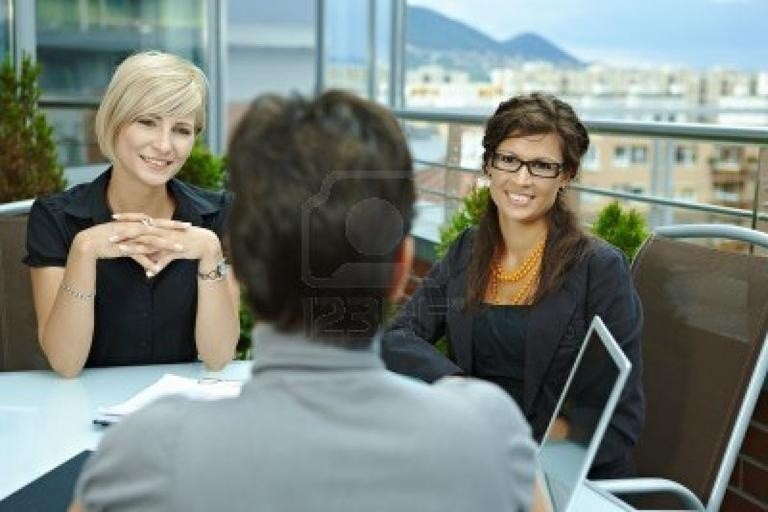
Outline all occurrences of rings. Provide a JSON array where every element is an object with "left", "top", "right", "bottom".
[{"left": 142, "top": 216, "right": 153, "bottom": 227}]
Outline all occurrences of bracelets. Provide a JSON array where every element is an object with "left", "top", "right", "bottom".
[
  {"left": 194, "top": 258, "right": 229, "bottom": 282},
  {"left": 55, "top": 282, "right": 95, "bottom": 303}
]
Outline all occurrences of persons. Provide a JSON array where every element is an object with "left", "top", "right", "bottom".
[
  {"left": 57, "top": 85, "right": 562, "bottom": 509},
  {"left": 17, "top": 49, "right": 242, "bottom": 384},
  {"left": 376, "top": 91, "right": 650, "bottom": 486}
]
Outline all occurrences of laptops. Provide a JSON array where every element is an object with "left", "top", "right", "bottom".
[{"left": 537, "top": 313, "right": 634, "bottom": 512}]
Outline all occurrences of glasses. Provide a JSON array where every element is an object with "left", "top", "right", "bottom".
[{"left": 487, "top": 151, "right": 569, "bottom": 179}]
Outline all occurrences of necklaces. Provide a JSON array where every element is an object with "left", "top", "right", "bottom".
[{"left": 488, "top": 228, "right": 549, "bottom": 308}]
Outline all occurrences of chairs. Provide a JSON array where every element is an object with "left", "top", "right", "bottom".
[{"left": 582, "top": 218, "right": 767, "bottom": 510}]
